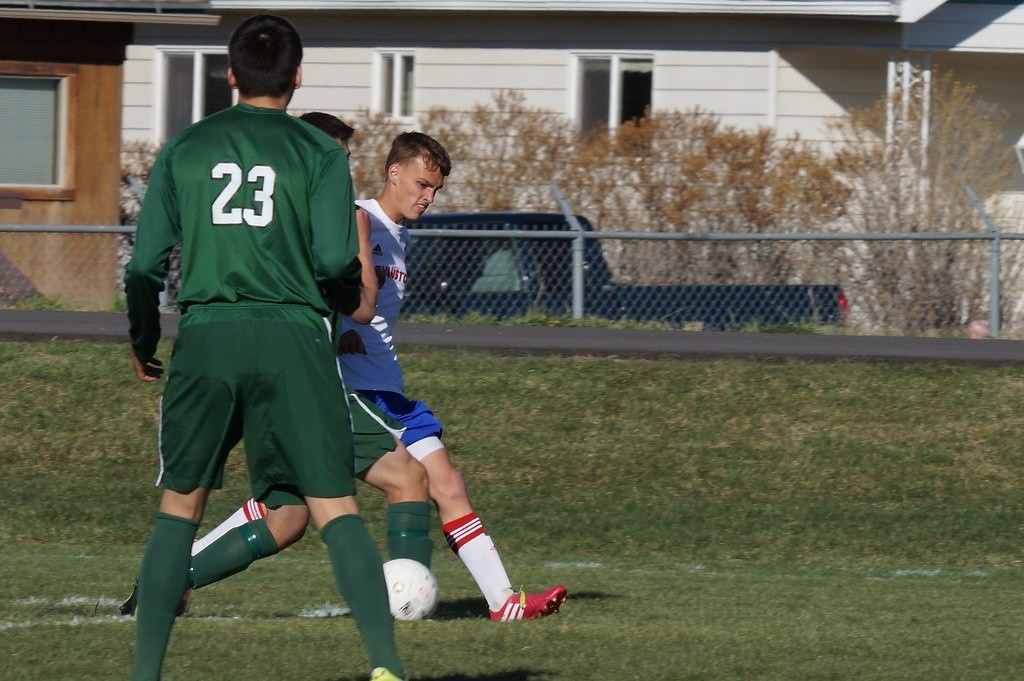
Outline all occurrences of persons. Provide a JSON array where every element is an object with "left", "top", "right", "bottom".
[
  {"left": 120, "top": 13, "right": 405, "bottom": 681},
  {"left": 121, "top": 112, "right": 436, "bottom": 622},
  {"left": 178, "top": 133, "right": 569, "bottom": 622}
]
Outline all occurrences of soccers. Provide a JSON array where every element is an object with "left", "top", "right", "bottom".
[{"left": 383, "top": 558, "right": 438, "bottom": 621}]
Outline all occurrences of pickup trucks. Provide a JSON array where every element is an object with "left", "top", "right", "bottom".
[{"left": 402, "top": 211, "right": 849, "bottom": 328}]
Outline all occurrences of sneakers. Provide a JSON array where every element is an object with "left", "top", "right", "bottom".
[
  {"left": 488, "top": 584, "right": 568, "bottom": 622},
  {"left": 119, "top": 579, "right": 189, "bottom": 616},
  {"left": 371, "top": 667, "right": 400, "bottom": 680}
]
